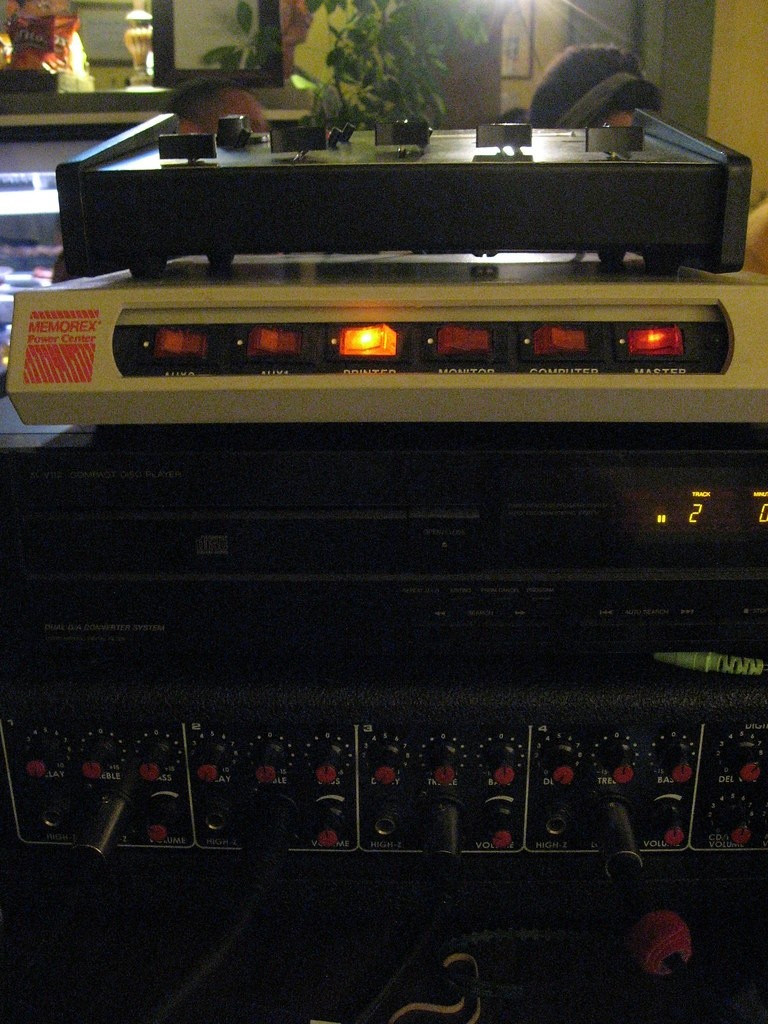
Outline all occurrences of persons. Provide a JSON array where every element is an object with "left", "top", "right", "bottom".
[
  {"left": 0, "top": 0, "right": 97, "bottom": 93},
  {"left": 531, "top": 44, "right": 662, "bottom": 129},
  {"left": 165, "top": 74, "right": 273, "bottom": 133}
]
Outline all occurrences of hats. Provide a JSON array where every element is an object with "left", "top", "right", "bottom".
[{"left": 530, "top": 44, "right": 661, "bottom": 131}]
